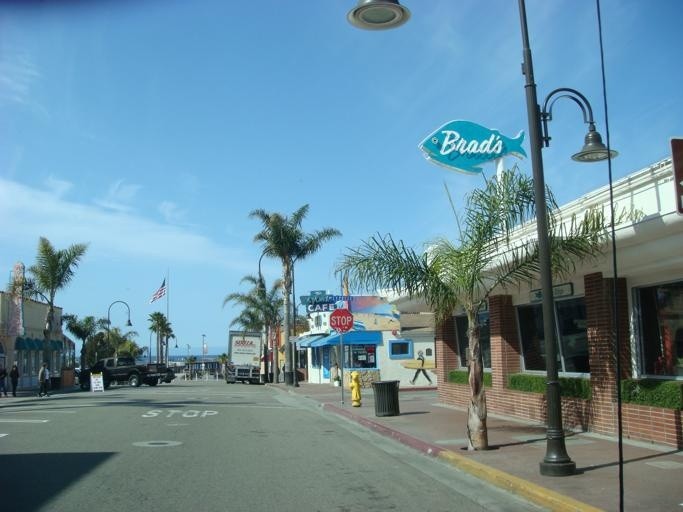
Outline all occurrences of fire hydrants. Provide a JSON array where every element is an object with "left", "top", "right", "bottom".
[{"left": 349, "top": 371, "right": 360, "bottom": 407}]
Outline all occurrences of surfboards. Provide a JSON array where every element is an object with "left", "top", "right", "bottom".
[{"left": 400, "top": 360, "right": 436, "bottom": 369}]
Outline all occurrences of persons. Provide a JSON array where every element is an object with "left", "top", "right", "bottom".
[
  {"left": 408, "top": 351, "right": 432, "bottom": 387},
  {"left": 8, "top": 365, "right": 19, "bottom": 397},
  {"left": 0, "top": 363, "right": 9, "bottom": 399},
  {"left": 37, "top": 362, "right": 51, "bottom": 397}
]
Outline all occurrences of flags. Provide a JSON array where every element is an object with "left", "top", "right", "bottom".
[{"left": 148, "top": 277, "right": 166, "bottom": 305}]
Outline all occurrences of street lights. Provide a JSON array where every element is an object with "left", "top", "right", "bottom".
[
  {"left": 345, "top": 0, "right": 617, "bottom": 475},
  {"left": 106, "top": 301, "right": 132, "bottom": 357},
  {"left": 165, "top": 334, "right": 177, "bottom": 364}
]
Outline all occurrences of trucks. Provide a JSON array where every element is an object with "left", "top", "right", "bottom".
[{"left": 222, "top": 331, "right": 261, "bottom": 384}]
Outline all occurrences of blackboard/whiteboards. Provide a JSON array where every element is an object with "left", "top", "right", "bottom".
[{"left": 91, "top": 373, "right": 104, "bottom": 390}]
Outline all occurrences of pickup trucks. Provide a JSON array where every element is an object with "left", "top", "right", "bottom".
[{"left": 78, "top": 357, "right": 160, "bottom": 391}]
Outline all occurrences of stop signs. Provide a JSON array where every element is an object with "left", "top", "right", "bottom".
[{"left": 329, "top": 309, "right": 352, "bottom": 333}]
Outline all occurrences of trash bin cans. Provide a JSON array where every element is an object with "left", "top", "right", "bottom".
[
  {"left": 371, "top": 380, "right": 400, "bottom": 416},
  {"left": 286, "top": 371, "right": 298, "bottom": 386},
  {"left": 269, "top": 373, "right": 277, "bottom": 382},
  {"left": 51, "top": 376, "right": 61, "bottom": 391},
  {"left": 261, "top": 374, "right": 268, "bottom": 383}
]
